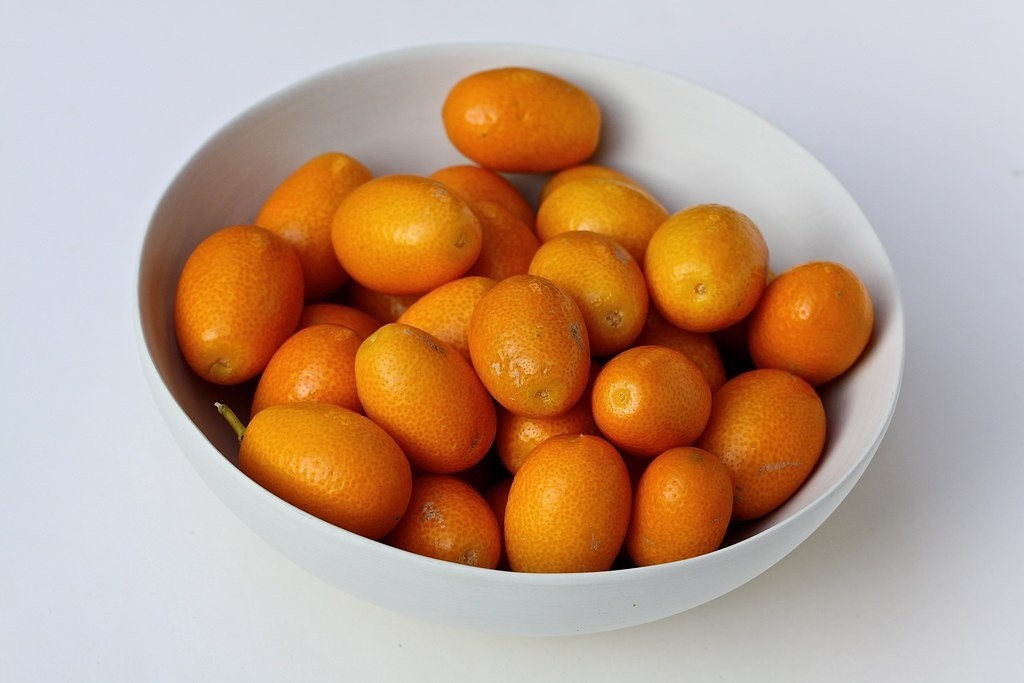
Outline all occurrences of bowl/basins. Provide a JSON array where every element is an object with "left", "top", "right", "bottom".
[{"left": 132, "top": 40, "right": 905, "bottom": 637}]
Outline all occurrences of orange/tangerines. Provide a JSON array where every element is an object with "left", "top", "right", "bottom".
[{"left": 177, "top": 67, "right": 872, "bottom": 573}]
